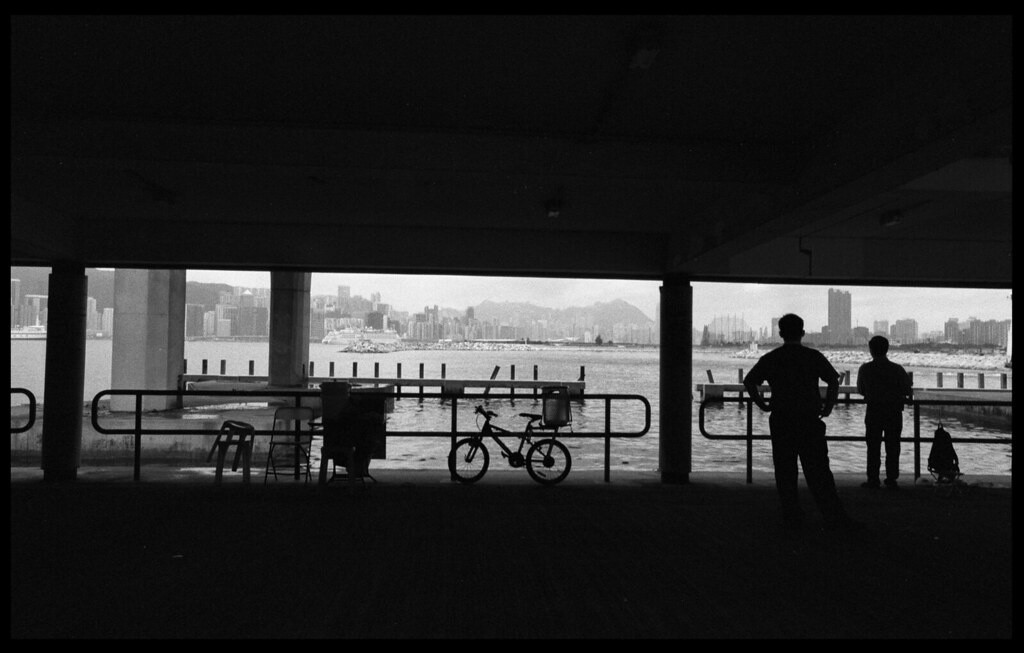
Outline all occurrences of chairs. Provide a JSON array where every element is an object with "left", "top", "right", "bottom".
[{"left": 264, "top": 407, "right": 315, "bottom": 486}]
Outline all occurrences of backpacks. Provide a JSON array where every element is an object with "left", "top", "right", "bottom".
[{"left": 927, "top": 422, "right": 960, "bottom": 483}]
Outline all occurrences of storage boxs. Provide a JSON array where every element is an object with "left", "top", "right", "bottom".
[{"left": 542, "top": 386, "right": 571, "bottom": 427}]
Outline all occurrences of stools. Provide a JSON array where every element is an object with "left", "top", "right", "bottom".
[{"left": 206, "top": 421, "right": 254, "bottom": 487}]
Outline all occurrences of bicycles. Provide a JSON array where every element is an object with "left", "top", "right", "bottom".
[{"left": 447, "top": 404, "right": 572, "bottom": 485}]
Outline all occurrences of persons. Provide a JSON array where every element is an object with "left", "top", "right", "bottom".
[
  {"left": 856, "top": 336, "right": 912, "bottom": 487},
  {"left": 744, "top": 313, "right": 840, "bottom": 500}
]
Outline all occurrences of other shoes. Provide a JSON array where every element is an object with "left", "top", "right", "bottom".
[
  {"left": 827, "top": 516, "right": 862, "bottom": 530},
  {"left": 861, "top": 481, "right": 880, "bottom": 489},
  {"left": 785, "top": 506, "right": 805, "bottom": 532},
  {"left": 884, "top": 478, "right": 898, "bottom": 487}
]
